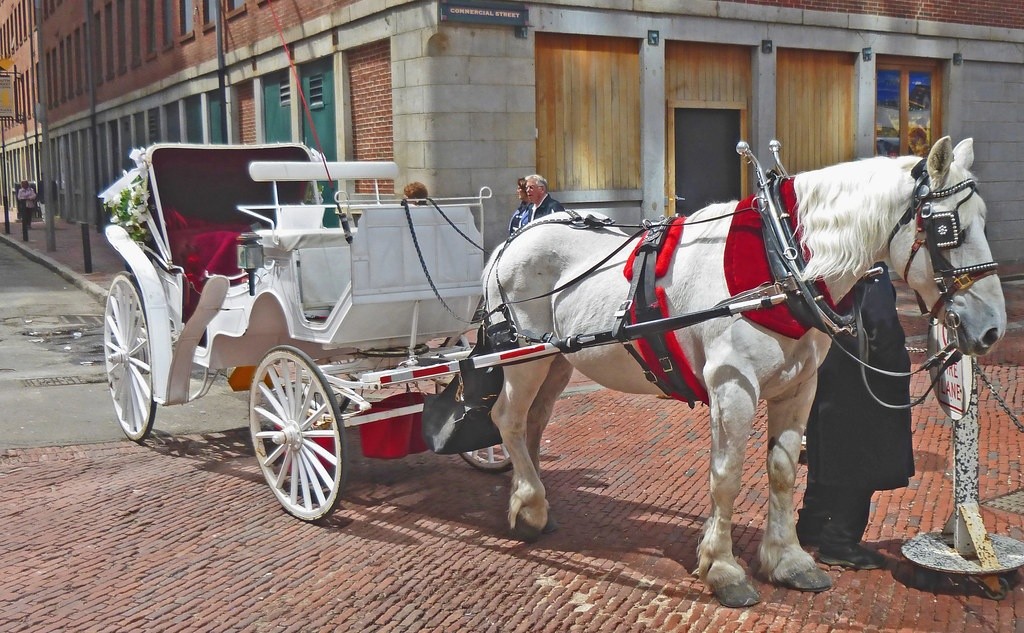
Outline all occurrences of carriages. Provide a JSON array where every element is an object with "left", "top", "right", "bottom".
[{"left": 103, "top": 134, "right": 1008, "bottom": 610}]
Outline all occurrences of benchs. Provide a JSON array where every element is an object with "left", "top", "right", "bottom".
[
  {"left": 147, "top": 140, "right": 318, "bottom": 325},
  {"left": 236, "top": 160, "right": 404, "bottom": 311}
]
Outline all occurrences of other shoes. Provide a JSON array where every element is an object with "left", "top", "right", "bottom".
[{"left": 816, "top": 539, "right": 887, "bottom": 570}]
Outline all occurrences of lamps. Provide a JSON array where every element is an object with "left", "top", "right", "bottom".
[{"left": 424, "top": 30, "right": 451, "bottom": 58}]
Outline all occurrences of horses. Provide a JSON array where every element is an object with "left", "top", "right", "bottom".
[{"left": 482, "top": 136, "right": 1007, "bottom": 608}]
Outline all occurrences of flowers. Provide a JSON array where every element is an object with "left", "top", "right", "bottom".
[{"left": 104, "top": 148, "right": 154, "bottom": 243}]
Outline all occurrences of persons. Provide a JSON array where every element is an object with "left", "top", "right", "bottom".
[
  {"left": 508, "top": 174, "right": 565, "bottom": 237},
  {"left": 794, "top": 260, "right": 914, "bottom": 565},
  {"left": 18, "top": 180, "right": 37, "bottom": 241}
]
